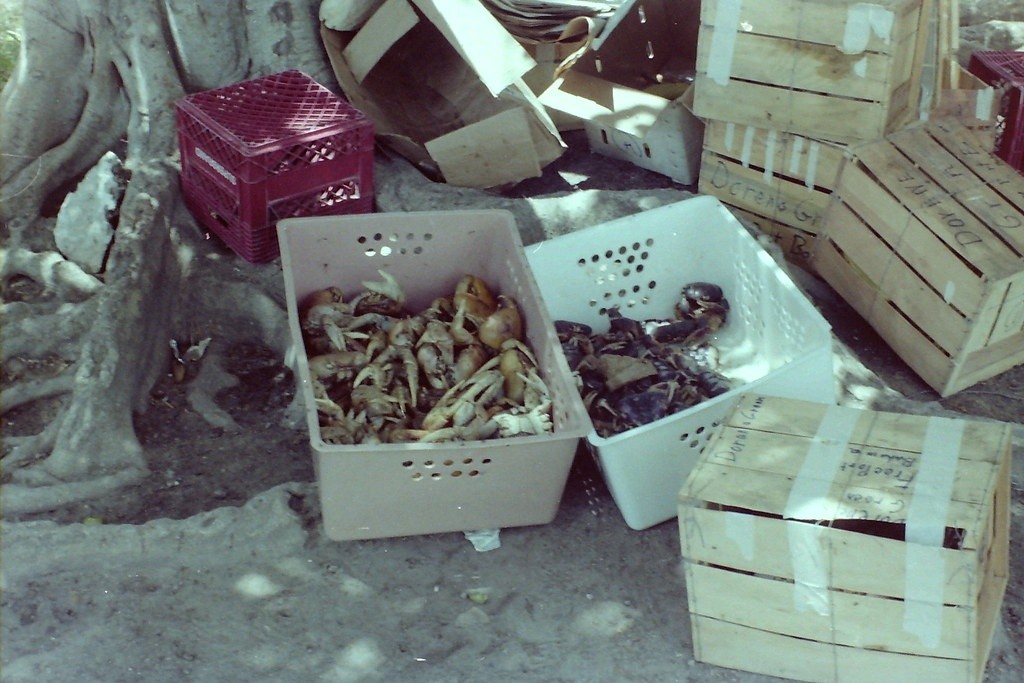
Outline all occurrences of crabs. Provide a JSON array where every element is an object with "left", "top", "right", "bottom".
[
  {"left": 298, "top": 266, "right": 551, "bottom": 441},
  {"left": 553, "top": 282, "right": 747, "bottom": 437}
]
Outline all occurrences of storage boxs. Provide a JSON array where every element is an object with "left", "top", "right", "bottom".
[
  {"left": 274, "top": 208, "right": 592, "bottom": 543},
  {"left": 172, "top": 67, "right": 375, "bottom": 266},
  {"left": 320, "top": 1, "right": 570, "bottom": 194},
  {"left": 692, "top": 2, "right": 929, "bottom": 141},
  {"left": 696, "top": 117, "right": 849, "bottom": 279},
  {"left": 523, "top": 193, "right": 839, "bottom": 531},
  {"left": 812, "top": 115, "right": 1024, "bottom": 398},
  {"left": 536, "top": 0, "right": 702, "bottom": 185},
  {"left": 675, "top": 393, "right": 1013, "bottom": 682},
  {"left": 968, "top": 50, "right": 1024, "bottom": 178}
]
